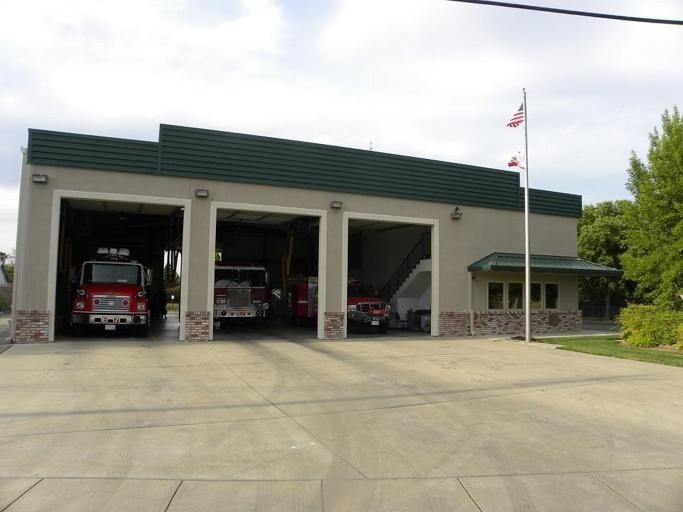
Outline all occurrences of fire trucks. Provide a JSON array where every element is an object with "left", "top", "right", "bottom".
[
  {"left": 67, "top": 247, "right": 166, "bottom": 336},
  {"left": 288, "top": 274, "right": 391, "bottom": 333},
  {"left": 214, "top": 260, "right": 270, "bottom": 319}
]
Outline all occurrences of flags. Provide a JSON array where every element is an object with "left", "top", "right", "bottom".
[{"left": 506, "top": 103, "right": 524, "bottom": 126}]
